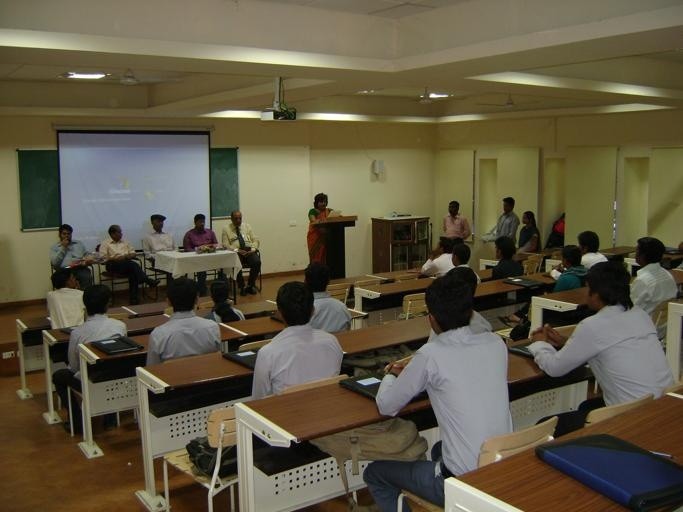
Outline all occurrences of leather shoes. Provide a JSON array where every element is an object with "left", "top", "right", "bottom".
[
  {"left": 248, "top": 285, "right": 256, "bottom": 294},
  {"left": 241, "top": 287, "right": 247, "bottom": 296},
  {"left": 148, "top": 279, "right": 160, "bottom": 287}
]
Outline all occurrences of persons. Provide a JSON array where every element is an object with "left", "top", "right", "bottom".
[
  {"left": 442, "top": 200, "right": 470, "bottom": 243},
  {"left": 221, "top": 210, "right": 261, "bottom": 296},
  {"left": 98, "top": 224, "right": 160, "bottom": 304},
  {"left": 204, "top": 281, "right": 245, "bottom": 322},
  {"left": 497, "top": 244, "right": 590, "bottom": 325},
  {"left": 575, "top": 230, "right": 609, "bottom": 271},
  {"left": 301, "top": 261, "right": 351, "bottom": 337},
  {"left": 49, "top": 223, "right": 91, "bottom": 291},
  {"left": 145, "top": 276, "right": 220, "bottom": 367},
  {"left": 491, "top": 236, "right": 524, "bottom": 280},
  {"left": 544, "top": 213, "right": 565, "bottom": 249},
  {"left": 444, "top": 244, "right": 481, "bottom": 287},
  {"left": 45, "top": 268, "right": 86, "bottom": 330},
  {"left": 141, "top": 215, "right": 177, "bottom": 263},
  {"left": 627, "top": 236, "right": 678, "bottom": 316},
  {"left": 362, "top": 274, "right": 514, "bottom": 511},
  {"left": 305, "top": 192, "right": 333, "bottom": 272},
  {"left": 422, "top": 235, "right": 456, "bottom": 279},
  {"left": 249, "top": 280, "right": 344, "bottom": 398},
  {"left": 50, "top": 285, "right": 128, "bottom": 434},
  {"left": 490, "top": 197, "right": 520, "bottom": 248},
  {"left": 182, "top": 214, "right": 218, "bottom": 283},
  {"left": 518, "top": 210, "right": 541, "bottom": 252},
  {"left": 526, "top": 260, "right": 678, "bottom": 441}
]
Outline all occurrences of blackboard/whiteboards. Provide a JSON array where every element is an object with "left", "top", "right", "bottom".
[{"left": 16, "top": 146, "right": 240, "bottom": 233}]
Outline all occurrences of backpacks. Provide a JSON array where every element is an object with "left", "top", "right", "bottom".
[
  {"left": 312, "top": 418, "right": 428, "bottom": 512},
  {"left": 188, "top": 436, "right": 239, "bottom": 476}
]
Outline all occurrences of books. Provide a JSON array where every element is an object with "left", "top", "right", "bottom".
[
  {"left": 508, "top": 341, "right": 534, "bottom": 357},
  {"left": 90, "top": 338, "right": 136, "bottom": 354},
  {"left": 338, "top": 372, "right": 387, "bottom": 399},
  {"left": 59, "top": 325, "right": 78, "bottom": 335},
  {"left": 221, "top": 347, "right": 260, "bottom": 369},
  {"left": 662, "top": 246, "right": 679, "bottom": 255},
  {"left": 500, "top": 275, "right": 542, "bottom": 289}
]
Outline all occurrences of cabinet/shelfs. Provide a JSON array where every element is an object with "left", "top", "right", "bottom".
[{"left": 371, "top": 217, "right": 433, "bottom": 273}]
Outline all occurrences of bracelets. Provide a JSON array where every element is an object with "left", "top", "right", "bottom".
[{"left": 388, "top": 372, "right": 397, "bottom": 377}]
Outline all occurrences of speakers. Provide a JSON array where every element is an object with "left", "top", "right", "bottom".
[{"left": 373, "top": 159, "right": 384, "bottom": 174}]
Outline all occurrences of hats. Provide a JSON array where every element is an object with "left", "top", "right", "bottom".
[{"left": 151, "top": 214, "right": 165, "bottom": 220}]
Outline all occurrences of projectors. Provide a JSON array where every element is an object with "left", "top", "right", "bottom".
[{"left": 260, "top": 110, "right": 297, "bottom": 122}]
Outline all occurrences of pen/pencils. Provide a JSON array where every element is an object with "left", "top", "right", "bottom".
[{"left": 650, "top": 450, "right": 673, "bottom": 458}]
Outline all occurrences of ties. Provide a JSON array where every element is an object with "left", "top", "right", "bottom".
[{"left": 236, "top": 227, "right": 246, "bottom": 250}]
